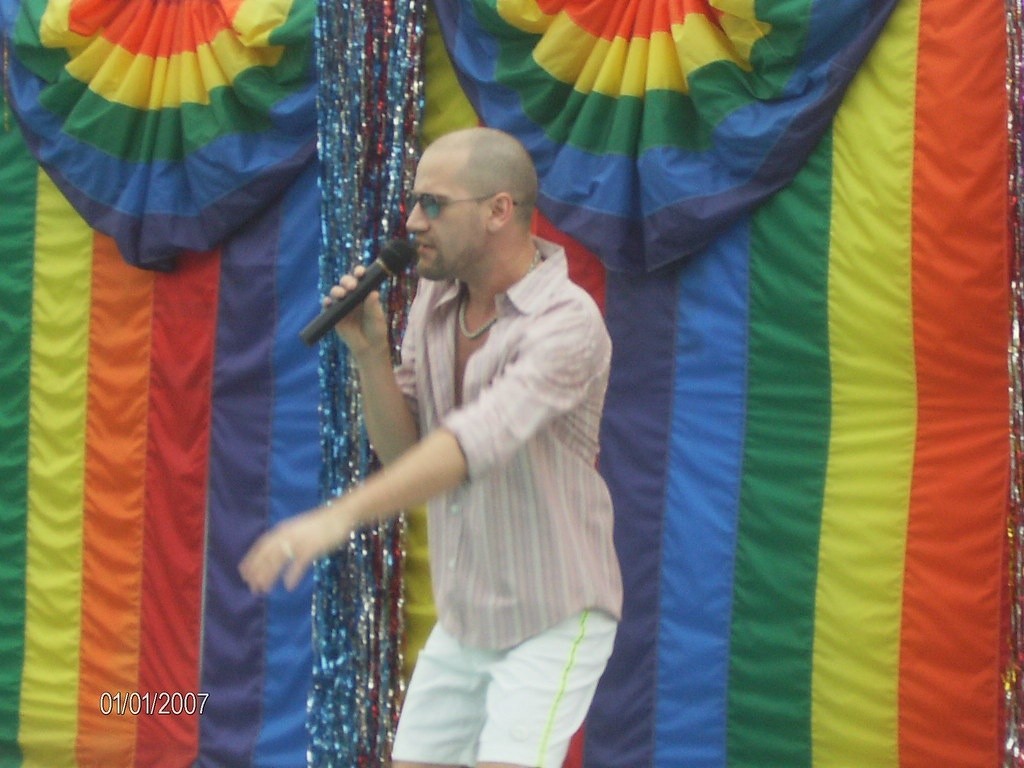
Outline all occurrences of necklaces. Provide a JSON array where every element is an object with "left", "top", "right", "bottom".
[{"left": 459, "top": 249, "right": 541, "bottom": 339}]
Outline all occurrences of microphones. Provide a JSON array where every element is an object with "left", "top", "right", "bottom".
[{"left": 300, "top": 241, "right": 412, "bottom": 346}]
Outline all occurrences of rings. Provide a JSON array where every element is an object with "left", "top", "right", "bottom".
[{"left": 281, "top": 545, "right": 292, "bottom": 560}]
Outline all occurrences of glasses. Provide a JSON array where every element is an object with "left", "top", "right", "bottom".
[{"left": 401, "top": 189, "right": 518, "bottom": 218}]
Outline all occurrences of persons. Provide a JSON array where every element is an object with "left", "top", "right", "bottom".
[{"left": 238, "top": 127, "right": 622, "bottom": 768}]
